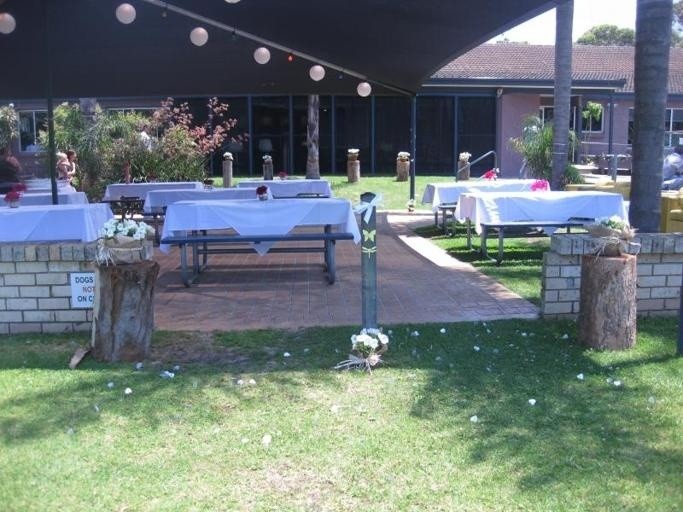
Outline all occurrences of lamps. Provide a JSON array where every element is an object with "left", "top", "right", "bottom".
[
  {"left": 0, "top": 10, "right": 16, "bottom": 39},
  {"left": 114, "top": 1, "right": 373, "bottom": 101}
]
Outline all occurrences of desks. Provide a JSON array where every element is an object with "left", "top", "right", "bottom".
[
  {"left": 423, "top": 179, "right": 629, "bottom": 264},
  {"left": 0, "top": 179, "right": 112, "bottom": 241},
  {"left": 104, "top": 180, "right": 361, "bottom": 282}
]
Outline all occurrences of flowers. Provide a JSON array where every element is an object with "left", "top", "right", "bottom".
[
  {"left": 333, "top": 327, "right": 392, "bottom": 377},
  {"left": 222, "top": 146, "right": 471, "bottom": 163}
]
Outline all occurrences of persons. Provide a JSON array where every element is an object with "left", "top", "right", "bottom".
[
  {"left": 67, "top": 149, "right": 77, "bottom": 185},
  {"left": 57, "top": 149, "right": 70, "bottom": 178},
  {"left": 663, "top": 146, "right": 683, "bottom": 190}
]
